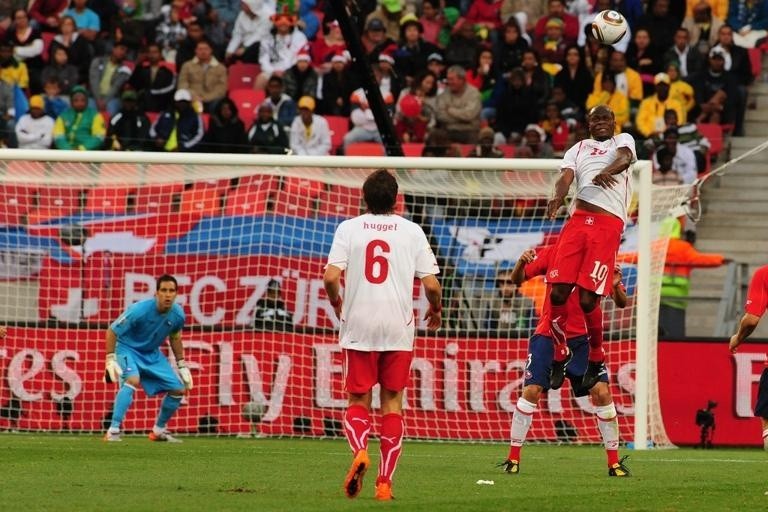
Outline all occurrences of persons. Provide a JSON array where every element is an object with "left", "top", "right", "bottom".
[
  {"left": 630, "top": 220, "right": 730, "bottom": 341},
  {"left": 486, "top": 270, "right": 537, "bottom": 340},
  {"left": 504, "top": 240, "right": 631, "bottom": 479},
  {"left": 0, "top": 2, "right": 767, "bottom": 232},
  {"left": 324, "top": 170, "right": 443, "bottom": 505},
  {"left": 104, "top": 271, "right": 194, "bottom": 443},
  {"left": 730, "top": 261, "right": 767, "bottom": 466},
  {"left": 255, "top": 279, "right": 292, "bottom": 327},
  {"left": 544, "top": 106, "right": 638, "bottom": 391}
]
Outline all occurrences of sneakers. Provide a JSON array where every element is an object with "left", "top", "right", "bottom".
[
  {"left": 505, "top": 459, "right": 520, "bottom": 473},
  {"left": 608, "top": 462, "right": 632, "bottom": 478},
  {"left": 148, "top": 429, "right": 183, "bottom": 443},
  {"left": 103, "top": 431, "right": 121, "bottom": 442},
  {"left": 581, "top": 359, "right": 605, "bottom": 390},
  {"left": 549, "top": 348, "right": 574, "bottom": 390},
  {"left": 374, "top": 483, "right": 394, "bottom": 501},
  {"left": 343, "top": 449, "right": 370, "bottom": 499}
]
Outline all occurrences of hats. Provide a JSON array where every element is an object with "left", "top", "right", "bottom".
[
  {"left": 295, "top": 46, "right": 313, "bottom": 63},
  {"left": 383, "top": 0, "right": 404, "bottom": 15},
  {"left": 367, "top": 18, "right": 386, "bottom": 32},
  {"left": 426, "top": 53, "right": 443, "bottom": 65},
  {"left": 298, "top": 96, "right": 317, "bottom": 112},
  {"left": 29, "top": 94, "right": 44, "bottom": 110},
  {"left": 378, "top": 45, "right": 398, "bottom": 66},
  {"left": 653, "top": 71, "right": 671, "bottom": 86},
  {"left": 174, "top": 88, "right": 191, "bottom": 102},
  {"left": 121, "top": 90, "right": 137, "bottom": 102},
  {"left": 331, "top": 49, "right": 347, "bottom": 65},
  {"left": 399, "top": 13, "right": 424, "bottom": 33}
]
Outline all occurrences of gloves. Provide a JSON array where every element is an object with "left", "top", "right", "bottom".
[
  {"left": 177, "top": 358, "right": 194, "bottom": 390},
  {"left": 102, "top": 352, "right": 123, "bottom": 384}
]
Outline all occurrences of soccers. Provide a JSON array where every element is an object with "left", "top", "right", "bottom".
[{"left": 590, "top": 9, "right": 627, "bottom": 45}]
